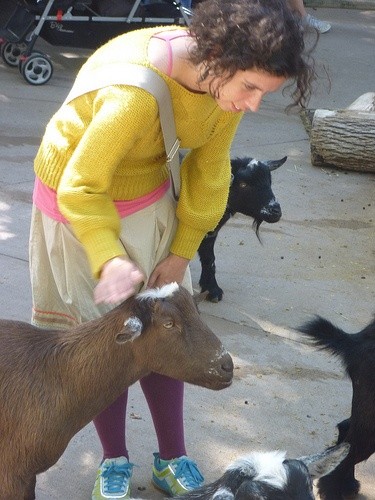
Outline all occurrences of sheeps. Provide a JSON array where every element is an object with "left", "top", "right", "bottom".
[
  {"left": 180, "top": 155, "right": 288, "bottom": 302},
  {"left": 163, "top": 441, "right": 351, "bottom": 500},
  {"left": 296, "top": 314, "right": 375, "bottom": 500},
  {"left": 0, "top": 282, "right": 234, "bottom": 500}
]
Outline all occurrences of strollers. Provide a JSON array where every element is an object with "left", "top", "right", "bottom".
[{"left": 1, "top": 0, "right": 202, "bottom": 86}]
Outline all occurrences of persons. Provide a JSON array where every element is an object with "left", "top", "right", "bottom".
[
  {"left": 284, "top": 0, "right": 330, "bottom": 33},
  {"left": 28, "top": 1, "right": 322, "bottom": 500}
]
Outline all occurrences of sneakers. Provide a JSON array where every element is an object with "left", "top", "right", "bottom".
[
  {"left": 92, "top": 456, "right": 135, "bottom": 500},
  {"left": 292, "top": 14, "right": 331, "bottom": 34},
  {"left": 151, "top": 448, "right": 205, "bottom": 498}
]
